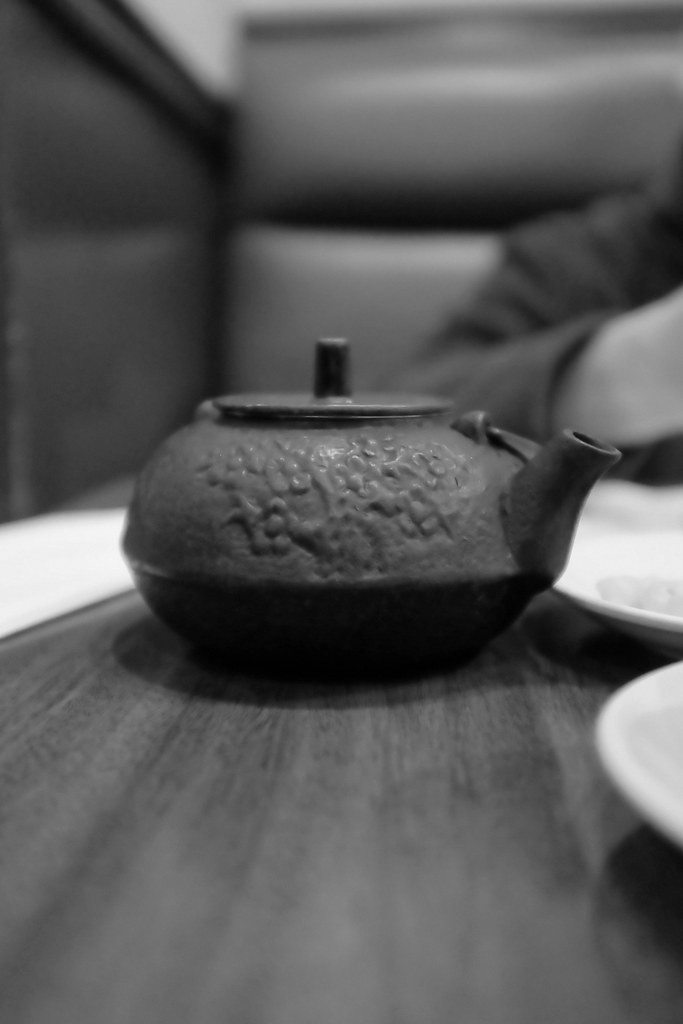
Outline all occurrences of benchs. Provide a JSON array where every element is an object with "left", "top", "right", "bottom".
[{"left": 227, "top": 16, "right": 682, "bottom": 405}]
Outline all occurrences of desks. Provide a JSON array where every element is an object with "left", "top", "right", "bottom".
[{"left": 0, "top": 471, "right": 683, "bottom": 1024}]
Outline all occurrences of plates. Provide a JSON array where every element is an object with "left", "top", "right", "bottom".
[
  {"left": 599, "top": 658, "right": 683, "bottom": 854},
  {"left": 547, "top": 477, "right": 683, "bottom": 656}
]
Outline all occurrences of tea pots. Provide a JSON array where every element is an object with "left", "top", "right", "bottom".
[{"left": 121, "top": 335, "right": 620, "bottom": 677}]
